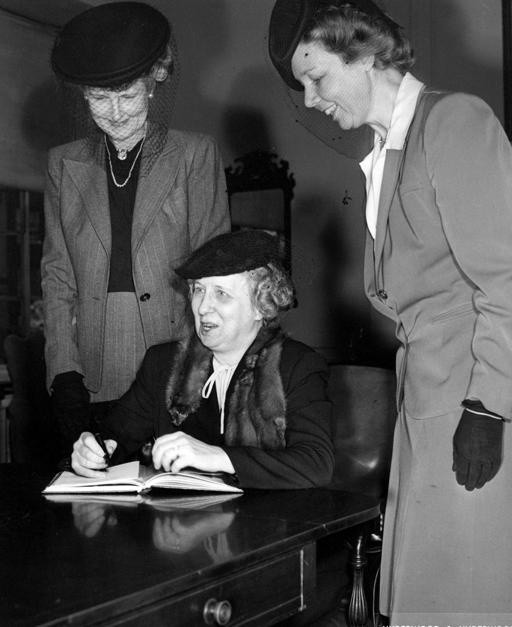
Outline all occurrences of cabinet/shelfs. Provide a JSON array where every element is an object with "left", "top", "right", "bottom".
[{"left": 1, "top": 183, "right": 43, "bottom": 386}]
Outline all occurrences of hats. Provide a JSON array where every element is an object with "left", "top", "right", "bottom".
[
  {"left": 268, "top": 1, "right": 309, "bottom": 91},
  {"left": 51, "top": 0, "right": 170, "bottom": 87},
  {"left": 174, "top": 231, "right": 278, "bottom": 279}
]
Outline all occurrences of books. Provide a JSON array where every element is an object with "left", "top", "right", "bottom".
[
  {"left": 40, "top": 458, "right": 243, "bottom": 493},
  {"left": 45, "top": 496, "right": 239, "bottom": 512}
]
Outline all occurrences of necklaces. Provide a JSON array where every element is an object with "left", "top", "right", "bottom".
[{"left": 104, "top": 133, "right": 146, "bottom": 188}]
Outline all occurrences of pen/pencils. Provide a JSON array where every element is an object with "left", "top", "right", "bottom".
[{"left": 90, "top": 414, "right": 113, "bottom": 465}]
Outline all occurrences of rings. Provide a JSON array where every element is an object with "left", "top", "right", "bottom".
[
  {"left": 167, "top": 446, "right": 180, "bottom": 456},
  {"left": 171, "top": 539, "right": 182, "bottom": 550}
]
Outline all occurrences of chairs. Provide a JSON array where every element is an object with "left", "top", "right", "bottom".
[
  {"left": 4, "top": 333, "right": 62, "bottom": 437},
  {"left": 328, "top": 365, "right": 396, "bottom": 627}
]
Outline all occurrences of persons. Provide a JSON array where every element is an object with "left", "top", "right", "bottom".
[
  {"left": 70, "top": 228, "right": 335, "bottom": 492},
  {"left": 71, "top": 501, "right": 239, "bottom": 556},
  {"left": 268, "top": 2, "right": 512, "bottom": 626},
  {"left": 39, "top": 2, "right": 232, "bottom": 429},
  {"left": 24, "top": 300, "right": 45, "bottom": 352}
]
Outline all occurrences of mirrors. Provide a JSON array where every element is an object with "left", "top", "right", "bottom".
[{"left": 224, "top": 141, "right": 298, "bottom": 311}]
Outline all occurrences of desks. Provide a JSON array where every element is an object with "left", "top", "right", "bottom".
[{"left": 0, "top": 460, "right": 380, "bottom": 627}]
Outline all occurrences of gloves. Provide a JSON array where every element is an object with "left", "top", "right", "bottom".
[
  {"left": 452, "top": 400, "right": 503, "bottom": 491},
  {"left": 52, "top": 375, "right": 90, "bottom": 430}
]
